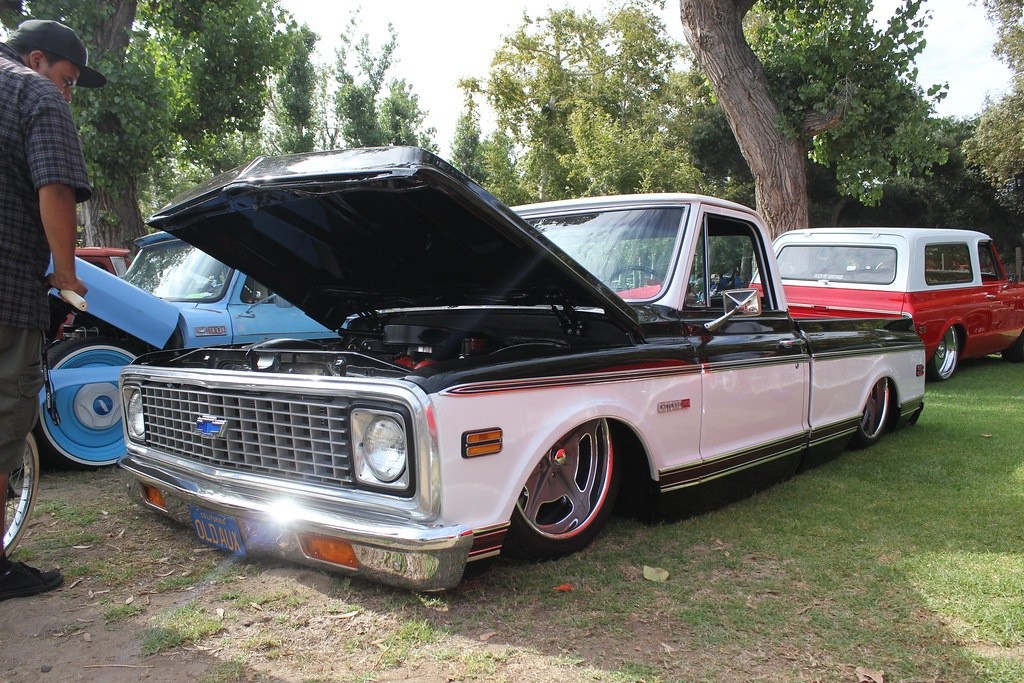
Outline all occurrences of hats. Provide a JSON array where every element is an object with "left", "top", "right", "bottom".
[{"left": 7, "top": 18, "right": 108, "bottom": 89}]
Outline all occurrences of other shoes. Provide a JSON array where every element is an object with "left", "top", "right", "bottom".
[{"left": 0, "top": 558, "right": 64, "bottom": 603}]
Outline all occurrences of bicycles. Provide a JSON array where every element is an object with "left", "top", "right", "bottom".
[{"left": 2, "top": 430, "right": 40, "bottom": 558}]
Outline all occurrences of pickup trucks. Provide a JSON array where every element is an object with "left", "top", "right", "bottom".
[{"left": 115, "top": 142, "right": 929, "bottom": 595}]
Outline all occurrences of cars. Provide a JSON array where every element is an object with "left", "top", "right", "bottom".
[
  {"left": 48, "top": 246, "right": 131, "bottom": 340},
  {"left": 37, "top": 228, "right": 370, "bottom": 471},
  {"left": 751, "top": 224, "right": 1024, "bottom": 381}
]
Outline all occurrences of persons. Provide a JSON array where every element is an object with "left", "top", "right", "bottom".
[{"left": 0, "top": 19, "right": 106, "bottom": 604}]
